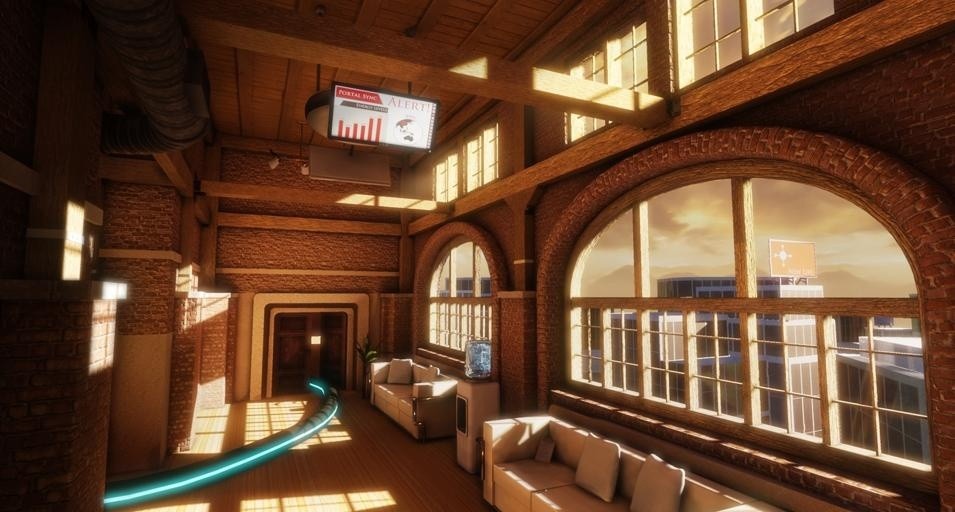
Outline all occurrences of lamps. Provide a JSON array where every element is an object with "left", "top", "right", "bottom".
[
  {"left": 300, "top": 161, "right": 311, "bottom": 177},
  {"left": 305, "top": 89, "right": 332, "bottom": 139},
  {"left": 266, "top": 148, "right": 282, "bottom": 170}
]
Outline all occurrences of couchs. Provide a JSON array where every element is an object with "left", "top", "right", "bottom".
[
  {"left": 370, "top": 360, "right": 459, "bottom": 443},
  {"left": 481, "top": 413, "right": 787, "bottom": 512}
]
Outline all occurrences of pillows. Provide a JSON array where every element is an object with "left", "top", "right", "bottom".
[
  {"left": 388, "top": 357, "right": 414, "bottom": 385},
  {"left": 573, "top": 430, "right": 687, "bottom": 511}
]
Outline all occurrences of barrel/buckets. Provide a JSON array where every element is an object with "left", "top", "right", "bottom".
[{"left": 464, "top": 340, "right": 491, "bottom": 378}]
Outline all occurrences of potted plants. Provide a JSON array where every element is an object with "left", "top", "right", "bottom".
[{"left": 353, "top": 334, "right": 380, "bottom": 399}]
